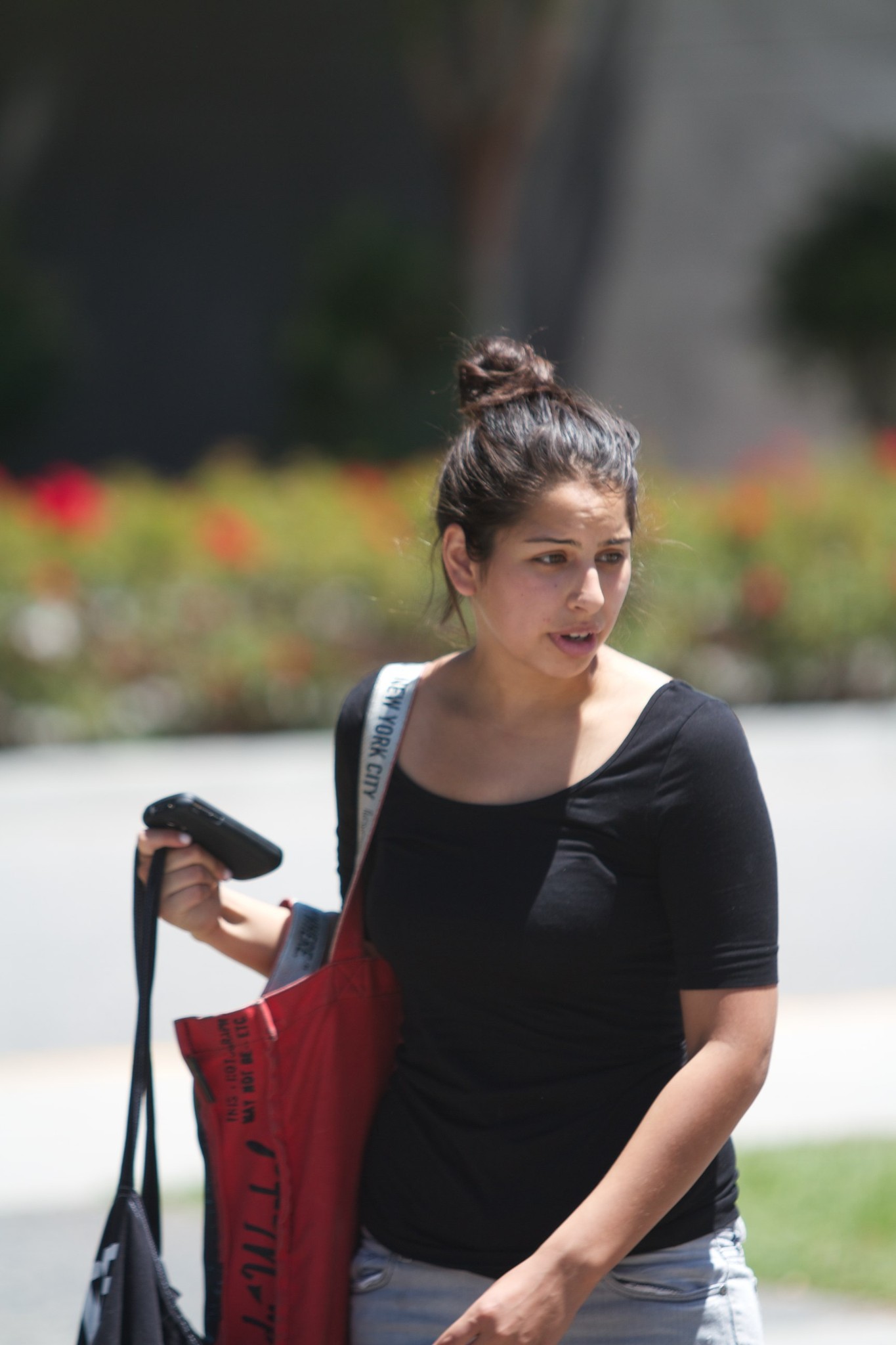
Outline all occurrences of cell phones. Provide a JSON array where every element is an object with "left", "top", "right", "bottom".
[{"left": 143, "top": 792, "right": 283, "bottom": 882}]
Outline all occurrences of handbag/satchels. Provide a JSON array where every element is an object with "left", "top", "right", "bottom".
[
  {"left": 78, "top": 1184, "right": 204, "bottom": 1345},
  {"left": 176, "top": 665, "right": 422, "bottom": 1345}
]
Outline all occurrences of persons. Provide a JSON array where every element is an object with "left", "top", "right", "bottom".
[{"left": 137, "top": 340, "right": 779, "bottom": 1345}]
